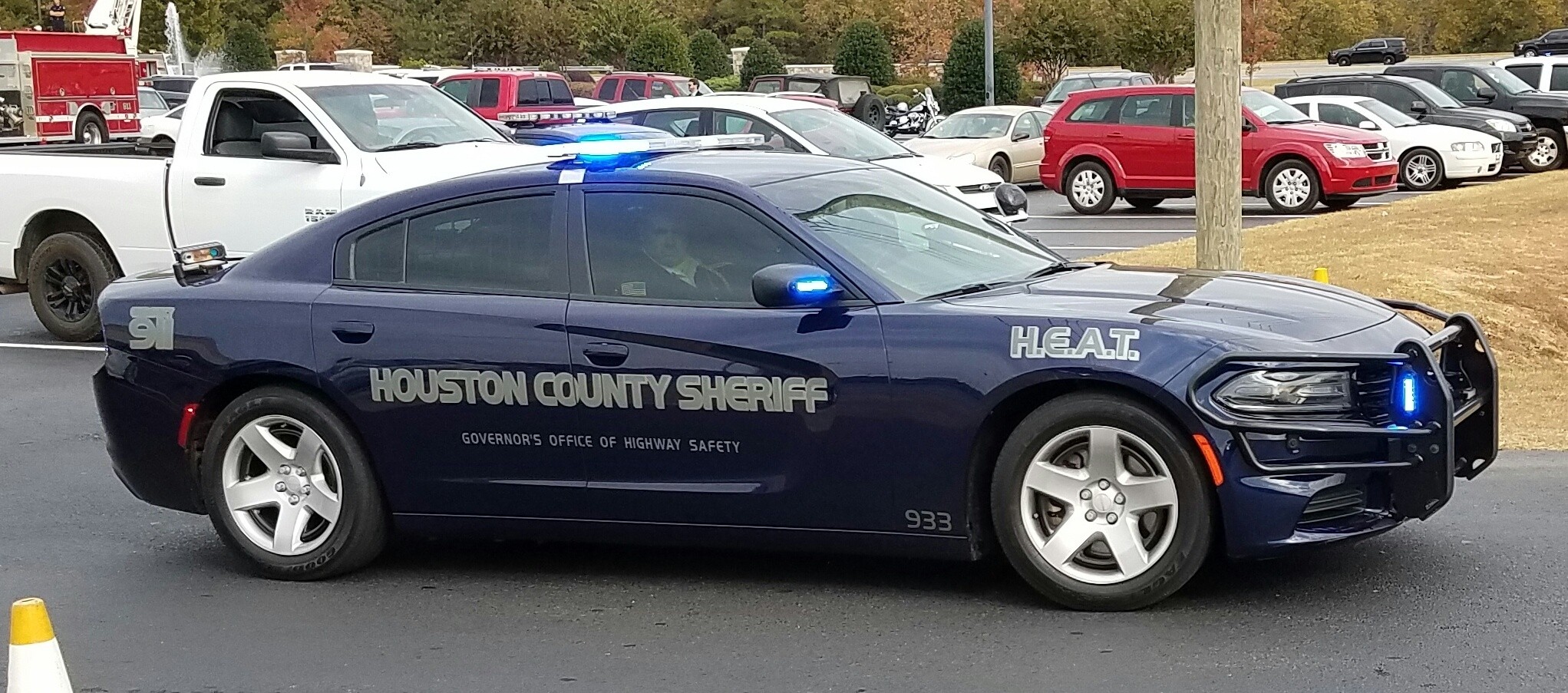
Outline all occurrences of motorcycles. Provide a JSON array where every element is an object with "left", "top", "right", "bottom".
[{"left": 883, "top": 87, "right": 948, "bottom": 135}]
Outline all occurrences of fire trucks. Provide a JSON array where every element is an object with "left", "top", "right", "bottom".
[{"left": 0, "top": 30, "right": 142, "bottom": 146}]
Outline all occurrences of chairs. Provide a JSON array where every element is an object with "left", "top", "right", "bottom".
[
  {"left": 210, "top": 107, "right": 263, "bottom": 156},
  {"left": 1443, "top": 72, "right": 1476, "bottom": 100},
  {"left": 1120, "top": 97, "right": 1138, "bottom": 125},
  {"left": 684, "top": 120, "right": 699, "bottom": 137},
  {"left": 1138, "top": 101, "right": 1163, "bottom": 125}
]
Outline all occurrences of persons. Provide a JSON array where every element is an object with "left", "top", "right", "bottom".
[
  {"left": 687, "top": 77, "right": 703, "bottom": 96},
  {"left": 616, "top": 196, "right": 729, "bottom": 303},
  {"left": 48, "top": 0, "right": 66, "bottom": 33},
  {"left": 352, "top": 104, "right": 392, "bottom": 148},
  {"left": 1464, "top": 74, "right": 1480, "bottom": 95}
]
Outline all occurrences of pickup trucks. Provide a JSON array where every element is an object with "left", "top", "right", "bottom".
[{"left": 1, "top": 70, "right": 561, "bottom": 346}]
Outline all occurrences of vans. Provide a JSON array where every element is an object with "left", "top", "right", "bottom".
[
  {"left": 431, "top": 69, "right": 578, "bottom": 126},
  {"left": 589, "top": 72, "right": 715, "bottom": 101}
]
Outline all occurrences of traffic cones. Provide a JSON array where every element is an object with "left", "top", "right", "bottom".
[{"left": 8, "top": 594, "right": 76, "bottom": 693}]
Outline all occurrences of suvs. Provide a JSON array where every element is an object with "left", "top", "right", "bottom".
[
  {"left": 1513, "top": 28, "right": 1568, "bottom": 57},
  {"left": 1271, "top": 57, "right": 1568, "bottom": 174},
  {"left": 1328, "top": 37, "right": 1410, "bottom": 66},
  {"left": 1038, "top": 84, "right": 1400, "bottom": 215},
  {"left": 746, "top": 74, "right": 886, "bottom": 134}
]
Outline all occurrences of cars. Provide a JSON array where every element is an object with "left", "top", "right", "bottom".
[
  {"left": 901, "top": 106, "right": 1054, "bottom": 188},
  {"left": 135, "top": 72, "right": 205, "bottom": 155},
  {"left": 1039, "top": 68, "right": 1159, "bottom": 122},
  {"left": 90, "top": 91, "right": 1503, "bottom": 614},
  {"left": 1255, "top": 95, "right": 1505, "bottom": 191}
]
